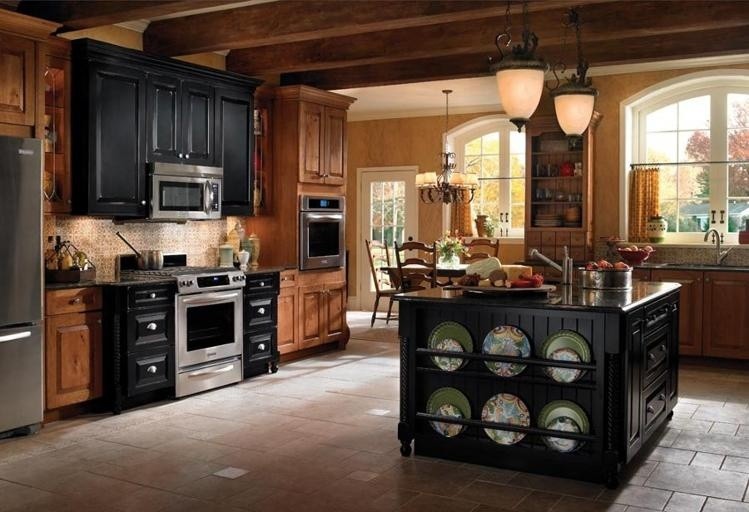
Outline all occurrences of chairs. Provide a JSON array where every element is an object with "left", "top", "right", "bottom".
[{"left": 362, "top": 231, "right": 501, "bottom": 331}]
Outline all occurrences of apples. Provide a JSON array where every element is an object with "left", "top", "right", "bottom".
[{"left": 489, "top": 270, "right": 506, "bottom": 286}]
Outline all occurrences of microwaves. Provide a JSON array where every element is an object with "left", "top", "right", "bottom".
[
  {"left": 300, "top": 212, "right": 345, "bottom": 269},
  {"left": 153, "top": 174, "right": 222, "bottom": 219}
]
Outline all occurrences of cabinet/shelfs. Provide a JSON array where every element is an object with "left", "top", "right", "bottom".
[
  {"left": 277, "top": 271, "right": 299, "bottom": 357},
  {"left": 244, "top": 271, "right": 281, "bottom": 378},
  {"left": 77, "top": 58, "right": 152, "bottom": 216},
  {"left": 299, "top": 268, "right": 345, "bottom": 353},
  {"left": 274, "top": 101, "right": 348, "bottom": 188},
  {"left": 250, "top": 93, "right": 275, "bottom": 218},
  {"left": 523, "top": 114, "right": 592, "bottom": 261},
  {"left": 148, "top": 68, "right": 220, "bottom": 164},
  {"left": 530, "top": 266, "right": 652, "bottom": 282},
  {"left": 45, "top": 285, "right": 111, "bottom": 419},
  {"left": 652, "top": 269, "right": 749, "bottom": 363},
  {"left": 214, "top": 79, "right": 254, "bottom": 216},
  {"left": 114, "top": 280, "right": 177, "bottom": 410},
  {"left": 0, "top": 34, "right": 35, "bottom": 130},
  {"left": 39, "top": 41, "right": 71, "bottom": 212}
]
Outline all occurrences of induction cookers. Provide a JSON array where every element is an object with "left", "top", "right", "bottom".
[{"left": 121, "top": 266, "right": 244, "bottom": 292}]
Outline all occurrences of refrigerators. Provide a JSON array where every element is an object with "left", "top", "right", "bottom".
[{"left": 1, "top": 133, "right": 44, "bottom": 437}]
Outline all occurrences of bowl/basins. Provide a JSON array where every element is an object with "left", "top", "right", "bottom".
[
  {"left": 534, "top": 188, "right": 555, "bottom": 201},
  {"left": 617, "top": 246, "right": 656, "bottom": 264},
  {"left": 564, "top": 207, "right": 580, "bottom": 222}
]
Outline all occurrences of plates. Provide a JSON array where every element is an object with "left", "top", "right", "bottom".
[
  {"left": 427, "top": 320, "right": 475, "bottom": 369},
  {"left": 563, "top": 222, "right": 581, "bottom": 228},
  {"left": 538, "top": 330, "right": 592, "bottom": 384},
  {"left": 555, "top": 192, "right": 581, "bottom": 201},
  {"left": 480, "top": 326, "right": 528, "bottom": 379},
  {"left": 478, "top": 395, "right": 531, "bottom": 444},
  {"left": 539, "top": 399, "right": 593, "bottom": 452},
  {"left": 424, "top": 386, "right": 472, "bottom": 439},
  {"left": 456, "top": 283, "right": 557, "bottom": 296},
  {"left": 535, "top": 214, "right": 563, "bottom": 227}
]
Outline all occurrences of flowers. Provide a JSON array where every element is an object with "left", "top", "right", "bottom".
[{"left": 483, "top": 217, "right": 495, "bottom": 234}]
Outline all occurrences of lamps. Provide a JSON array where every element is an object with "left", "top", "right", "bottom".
[
  {"left": 486, "top": 1, "right": 568, "bottom": 135},
  {"left": 416, "top": 89, "right": 478, "bottom": 205},
  {"left": 551, "top": 2, "right": 600, "bottom": 149}
]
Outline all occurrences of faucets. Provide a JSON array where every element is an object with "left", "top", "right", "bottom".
[
  {"left": 528, "top": 245, "right": 573, "bottom": 284},
  {"left": 703, "top": 228, "right": 734, "bottom": 266}
]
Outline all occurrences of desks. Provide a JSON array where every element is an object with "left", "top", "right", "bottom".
[{"left": 392, "top": 280, "right": 682, "bottom": 486}]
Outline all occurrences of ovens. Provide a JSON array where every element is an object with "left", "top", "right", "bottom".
[{"left": 174, "top": 290, "right": 244, "bottom": 398}]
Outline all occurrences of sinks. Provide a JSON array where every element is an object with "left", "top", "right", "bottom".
[
  {"left": 662, "top": 262, "right": 730, "bottom": 268},
  {"left": 542, "top": 278, "right": 561, "bottom": 286}
]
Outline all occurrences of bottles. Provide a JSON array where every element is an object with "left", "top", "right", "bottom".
[
  {"left": 535, "top": 157, "right": 560, "bottom": 178},
  {"left": 220, "top": 221, "right": 261, "bottom": 269},
  {"left": 647, "top": 216, "right": 666, "bottom": 243},
  {"left": 562, "top": 159, "right": 573, "bottom": 176}
]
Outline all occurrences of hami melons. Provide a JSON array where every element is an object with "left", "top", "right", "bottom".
[{"left": 465, "top": 257, "right": 501, "bottom": 281}]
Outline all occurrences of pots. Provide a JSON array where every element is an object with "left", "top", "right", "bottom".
[{"left": 115, "top": 231, "right": 164, "bottom": 271}]
